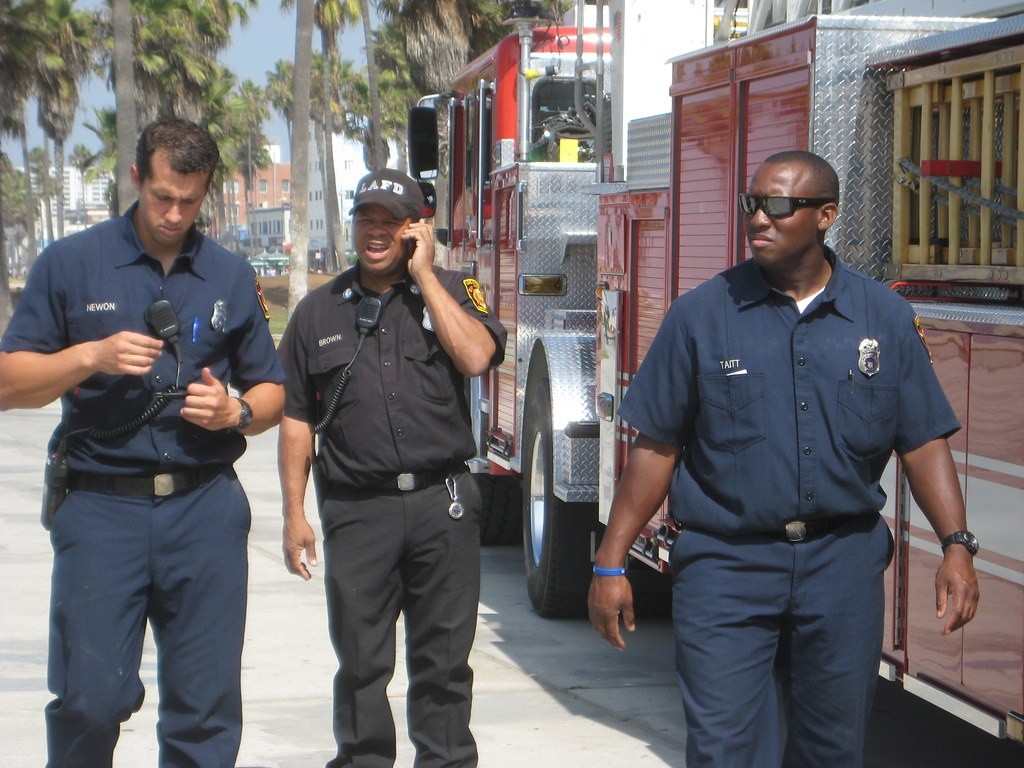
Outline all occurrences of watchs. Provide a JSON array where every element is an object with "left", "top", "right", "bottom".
[
  {"left": 228, "top": 397, "right": 251, "bottom": 432},
  {"left": 941, "top": 531, "right": 979, "bottom": 555}
]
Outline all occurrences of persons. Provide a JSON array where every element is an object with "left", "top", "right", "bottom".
[
  {"left": 0, "top": 118, "right": 285, "bottom": 768},
  {"left": 587, "top": 150, "right": 980, "bottom": 768},
  {"left": 278, "top": 170, "right": 507, "bottom": 768}
]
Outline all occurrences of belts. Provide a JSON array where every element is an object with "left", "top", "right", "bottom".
[
  {"left": 65, "top": 465, "right": 222, "bottom": 497},
  {"left": 764, "top": 519, "right": 844, "bottom": 543},
  {"left": 376, "top": 459, "right": 464, "bottom": 496}
]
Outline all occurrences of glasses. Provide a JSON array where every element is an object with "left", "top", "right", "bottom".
[{"left": 738, "top": 193, "right": 834, "bottom": 219}]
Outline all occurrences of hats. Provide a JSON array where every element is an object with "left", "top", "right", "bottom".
[{"left": 348, "top": 170, "right": 424, "bottom": 223}]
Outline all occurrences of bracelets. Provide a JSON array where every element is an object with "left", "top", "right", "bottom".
[{"left": 593, "top": 565, "right": 625, "bottom": 576}]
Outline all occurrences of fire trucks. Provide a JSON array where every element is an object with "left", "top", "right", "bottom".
[{"left": 403, "top": 0, "right": 1024, "bottom": 749}]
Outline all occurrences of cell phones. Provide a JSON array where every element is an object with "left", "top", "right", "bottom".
[{"left": 406, "top": 218, "right": 428, "bottom": 258}]
